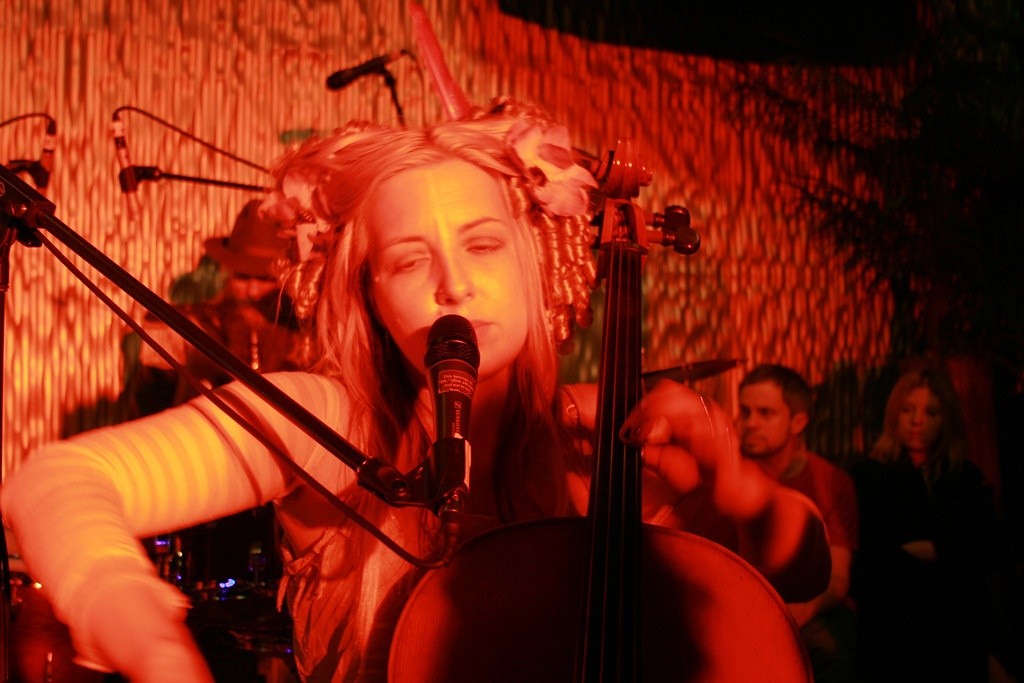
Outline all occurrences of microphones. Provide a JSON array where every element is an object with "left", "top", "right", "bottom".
[
  {"left": 423, "top": 314, "right": 481, "bottom": 544},
  {"left": 35, "top": 119, "right": 56, "bottom": 198},
  {"left": 324, "top": 49, "right": 405, "bottom": 91},
  {"left": 109, "top": 119, "right": 143, "bottom": 221}
]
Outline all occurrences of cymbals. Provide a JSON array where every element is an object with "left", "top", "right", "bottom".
[{"left": 642, "top": 357, "right": 743, "bottom": 388}]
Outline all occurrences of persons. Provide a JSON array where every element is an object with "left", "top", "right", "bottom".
[
  {"left": 850, "top": 367, "right": 1005, "bottom": 683},
  {"left": 730, "top": 358, "right": 858, "bottom": 683},
  {"left": 0, "top": 124, "right": 832, "bottom": 683}
]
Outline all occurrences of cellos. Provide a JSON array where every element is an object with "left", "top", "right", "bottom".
[{"left": 377, "top": 134, "right": 817, "bottom": 683}]
